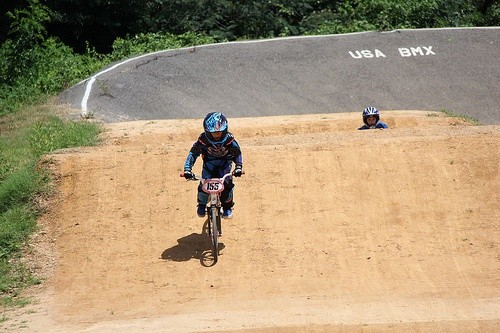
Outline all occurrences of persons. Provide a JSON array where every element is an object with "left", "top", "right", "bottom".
[
  {"left": 357, "top": 107, "right": 388, "bottom": 130},
  {"left": 184, "top": 112, "right": 242, "bottom": 218}
]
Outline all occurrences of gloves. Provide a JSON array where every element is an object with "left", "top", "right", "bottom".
[
  {"left": 184, "top": 169, "right": 195, "bottom": 181},
  {"left": 233, "top": 167, "right": 242, "bottom": 177}
]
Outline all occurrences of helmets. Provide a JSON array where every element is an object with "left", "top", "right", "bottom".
[
  {"left": 203, "top": 111, "right": 229, "bottom": 149},
  {"left": 362, "top": 106, "right": 380, "bottom": 128}
]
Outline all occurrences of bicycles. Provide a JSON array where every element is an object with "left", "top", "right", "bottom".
[{"left": 180, "top": 171, "right": 245, "bottom": 262}]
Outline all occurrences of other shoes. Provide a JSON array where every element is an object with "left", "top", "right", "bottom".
[
  {"left": 223, "top": 209, "right": 232, "bottom": 218},
  {"left": 196, "top": 204, "right": 205, "bottom": 216}
]
